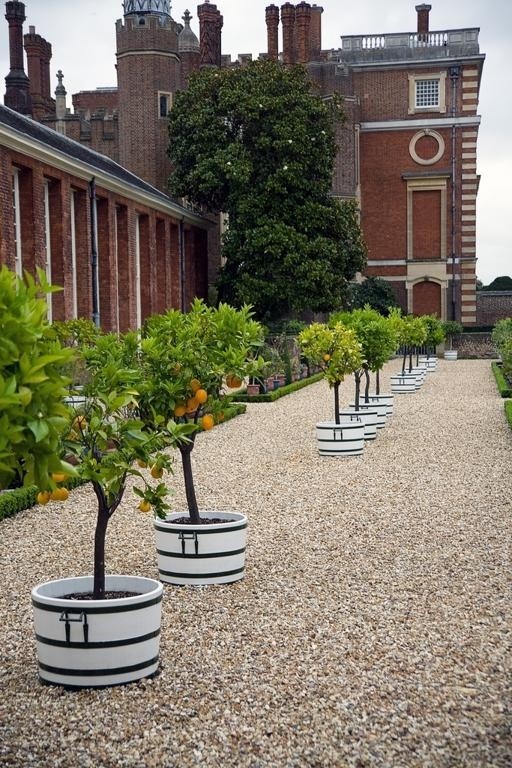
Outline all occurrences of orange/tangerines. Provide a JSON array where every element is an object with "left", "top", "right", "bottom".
[
  {"left": 37, "top": 400, "right": 164, "bottom": 513},
  {"left": 172, "top": 362, "right": 243, "bottom": 429},
  {"left": 323, "top": 353, "right": 330, "bottom": 360}
]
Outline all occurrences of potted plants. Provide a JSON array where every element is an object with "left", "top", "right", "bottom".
[
  {"left": 292, "top": 302, "right": 464, "bottom": 457},
  {"left": 134, "top": 296, "right": 274, "bottom": 586},
  {"left": 246, "top": 362, "right": 322, "bottom": 396},
  {"left": 18, "top": 326, "right": 200, "bottom": 690}
]
